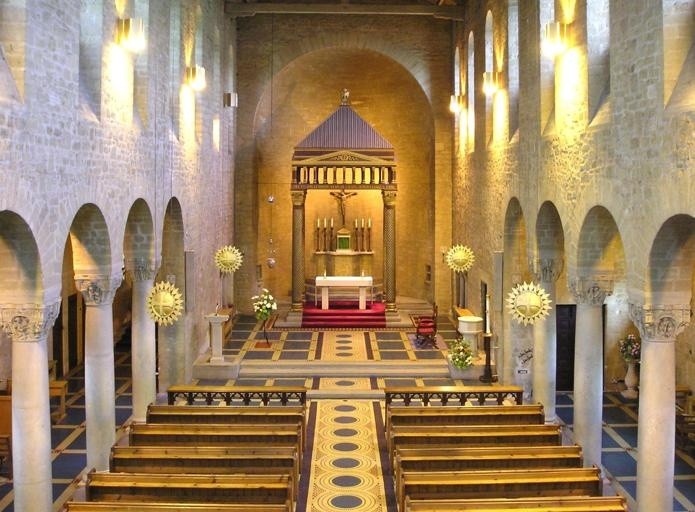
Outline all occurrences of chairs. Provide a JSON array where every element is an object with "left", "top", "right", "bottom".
[{"left": 417, "top": 302, "right": 439, "bottom": 349}]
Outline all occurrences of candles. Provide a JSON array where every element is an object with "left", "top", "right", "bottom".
[
  {"left": 324, "top": 216, "right": 327, "bottom": 228},
  {"left": 317, "top": 216, "right": 320, "bottom": 228},
  {"left": 354, "top": 217, "right": 357, "bottom": 228},
  {"left": 368, "top": 217, "right": 371, "bottom": 227},
  {"left": 330, "top": 216, "right": 334, "bottom": 227},
  {"left": 361, "top": 218, "right": 365, "bottom": 228},
  {"left": 324, "top": 266, "right": 326, "bottom": 278},
  {"left": 362, "top": 269, "right": 364, "bottom": 278}
]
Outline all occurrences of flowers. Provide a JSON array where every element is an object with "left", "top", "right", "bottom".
[
  {"left": 450, "top": 337, "right": 474, "bottom": 369},
  {"left": 252, "top": 288, "right": 278, "bottom": 320},
  {"left": 619, "top": 334, "right": 641, "bottom": 359}
]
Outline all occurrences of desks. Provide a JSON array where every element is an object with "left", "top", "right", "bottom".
[{"left": 4, "top": 378, "right": 69, "bottom": 421}]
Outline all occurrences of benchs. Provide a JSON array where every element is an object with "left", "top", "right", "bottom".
[
  {"left": 64, "top": 384, "right": 308, "bottom": 512},
  {"left": 384, "top": 385, "right": 631, "bottom": 511}
]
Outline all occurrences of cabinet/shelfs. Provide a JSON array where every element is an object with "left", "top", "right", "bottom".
[{"left": 209, "top": 307, "right": 233, "bottom": 347}]
